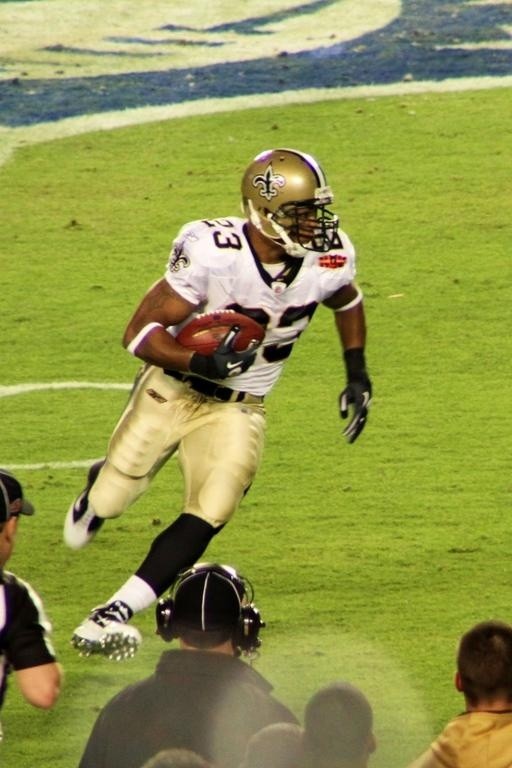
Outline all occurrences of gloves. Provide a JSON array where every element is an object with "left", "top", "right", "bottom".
[
  {"left": 207, "top": 325, "right": 259, "bottom": 381},
  {"left": 338, "top": 375, "right": 375, "bottom": 445}
]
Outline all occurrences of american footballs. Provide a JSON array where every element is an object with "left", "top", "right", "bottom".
[{"left": 176, "top": 309, "right": 262, "bottom": 358}]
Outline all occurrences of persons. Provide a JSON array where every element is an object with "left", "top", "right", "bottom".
[
  {"left": 78, "top": 563, "right": 376, "bottom": 767},
  {"left": 408, "top": 620, "right": 512, "bottom": 768},
  {"left": 0, "top": 467, "right": 63, "bottom": 720},
  {"left": 63, "top": 147, "right": 372, "bottom": 660}
]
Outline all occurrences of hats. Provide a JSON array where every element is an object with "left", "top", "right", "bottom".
[
  {"left": 0, "top": 463, "right": 36, "bottom": 530},
  {"left": 169, "top": 559, "right": 245, "bottom": 630}
]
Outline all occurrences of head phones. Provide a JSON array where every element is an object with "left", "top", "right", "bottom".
[{"left": 155, "top": 562, "right": 261, "bottom": 648}]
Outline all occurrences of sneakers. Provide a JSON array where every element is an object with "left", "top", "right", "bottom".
[
  {"left": 60, "top": 458, "right": 105, "bottom": 552},
  {"left": 67, "top": 597, "right": 143, "bottom": 662}
]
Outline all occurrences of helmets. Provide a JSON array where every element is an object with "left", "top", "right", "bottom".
[{"left": 240, "top": 145, "right": 346, "bottom": 258}]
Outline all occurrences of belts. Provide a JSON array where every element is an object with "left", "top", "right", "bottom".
[{"left": 163, "top": 369, "right": 267, "bottom": 404}]
